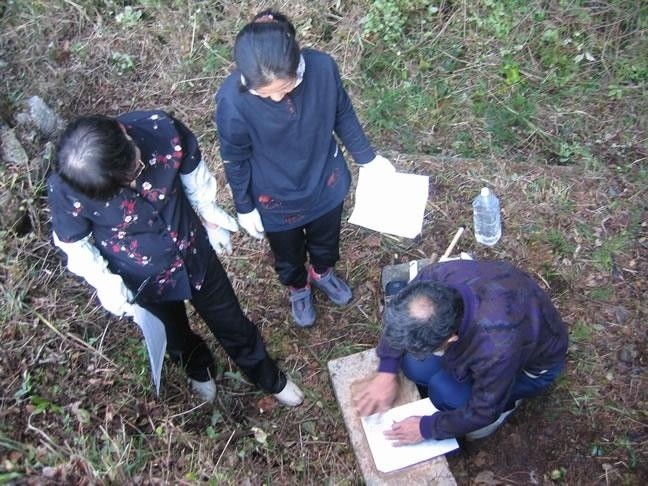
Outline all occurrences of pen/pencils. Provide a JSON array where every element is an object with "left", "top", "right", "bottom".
[{"left": 119, "top": 276, "right": 151, "bottom": 321}]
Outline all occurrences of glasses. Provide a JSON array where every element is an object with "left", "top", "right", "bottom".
[{"left": 133, "top": 160, "right": 146, "bottom": 180}]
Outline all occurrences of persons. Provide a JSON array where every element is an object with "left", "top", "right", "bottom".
[
  {"left": 215, "top": 7, "right": 397, "bottom": 328},
  {"left": 45, "top": 104, "right": 307, "bottom": 408},
  {"left": 350, "top": 259, "right": 570, "bottom": 441}
]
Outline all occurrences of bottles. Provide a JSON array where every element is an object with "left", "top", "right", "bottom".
[{"left": 472, "top": 187, "right": 502, "bottom": 247}]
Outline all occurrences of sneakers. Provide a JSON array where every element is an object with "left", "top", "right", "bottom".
[
  {"left": 464, "top": 396, "right": 523, "bottom": 441},
  {"left": 192, "top": 375, "right": 217, "bottom": 406},
  {"left": 288, "top": 285, "right": 317, "bottom": 328},
  {"left": 274, "top": 378, "right": 305, "bottom": 407},
  {"left": 308, "top": 264, "right": 353, "bottom": 306}
]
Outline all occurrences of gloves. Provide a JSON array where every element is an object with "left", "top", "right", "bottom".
[
  {"left": 362, "top": 156, "right": 396, "bottom": 174},
  {"left": 207, "top": 226, "right": 233, "bottom": 257},
  {"left": 237, "top": 209, "right": 265, "bottom": 242},
  {"left": 96, "top": 274, "right": 137, "bottom": 317}
]
